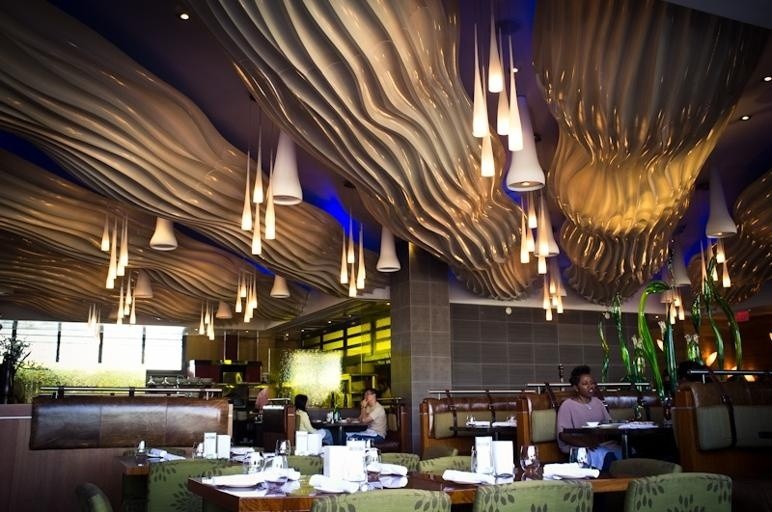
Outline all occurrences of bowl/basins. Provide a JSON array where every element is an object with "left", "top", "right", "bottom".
[{"left": 586, "top": 421, "right": 600, "bottom": 426}]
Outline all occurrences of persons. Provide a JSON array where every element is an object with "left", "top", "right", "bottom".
[
  {"left": 295, "top": 393, "right": 335, "bottom": 445},
  {"left": 557, "top": 365, "right": 636, "bottom": 473},
  {"left": 255, "top": 373, "right": 279, "bottom": 412},
  {"left": 345, "top": 388, "right": 387, "bottom": 445}
]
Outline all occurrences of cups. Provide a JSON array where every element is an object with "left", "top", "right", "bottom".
[
  {"left": 242, "top": 451, "right": 265, "bottom": 474},
  {"left": 520, "top": 444, "right": 540, "bottom": 473},
  {"left": 147, "top": 375, "right": 214, "bottom": 386},
  {"left": 191, "top": 441, "right": 204, "bottom": 459},
  {"left": 364, "top": 447, "right": 381, "bottom": 467},
  {"left": 466, "top": 414, "right": 476, "bottom": 426},
  {"left": 276, "top": 438, "right": 292, "bottom": 455},
  {"left": 133, "top": 440, "right": 150, "bottom": 458},
  {"left": 346, "top": 438, "right": 374, "bottom": 451},
  {"left": 261, "top": 457, "right": 288, "bottom": 490},
  {"left": 569, "top": 446, "right": 592, "bottom": 470}
]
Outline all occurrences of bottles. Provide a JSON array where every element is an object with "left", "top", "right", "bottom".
[{"left": 333, "top": 404, "right": 341, "bottom": 423}]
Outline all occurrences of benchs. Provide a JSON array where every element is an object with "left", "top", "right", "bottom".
[
  {"left": 0, "top": 387, "right": 234, "bottom": 511},
  {"left": 369, "top": 397, "right": 411, "bottom": 452},
  {"left": 674, "top": 370, "right": 772, "bottom": 512},
  {"left": 420, "top": 389, "right": 537, "bottom": 459},
  {"left": 516, "top": 382, "right": 664, "bottom": 465},
  {"left": 261, "top": 398, "right": 296, "bottom": 451}
]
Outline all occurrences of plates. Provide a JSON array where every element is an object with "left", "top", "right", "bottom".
[
  {"left": 224, "top": 485, "right": 255, "bottom": 488},
  {"left": 146, "top": 453, "right": 161, "bottom": 458},
  {"left": 314, "top": 486, "right": 344, "bottom": 494}
]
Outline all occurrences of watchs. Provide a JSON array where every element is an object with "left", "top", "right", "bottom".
[{"left": 360, "top": 405, "right": 367, "bottom": 409}]
